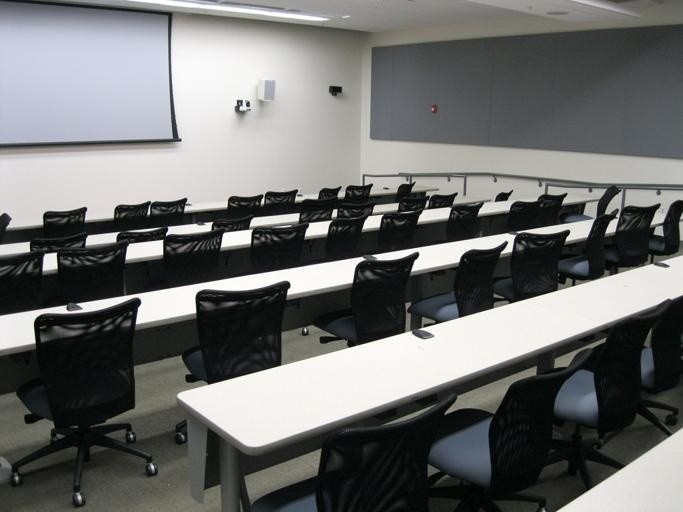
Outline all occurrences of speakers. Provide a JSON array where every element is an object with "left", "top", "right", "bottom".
[{"left": 258, "top": 79, "right": 277, "bottom": 101}]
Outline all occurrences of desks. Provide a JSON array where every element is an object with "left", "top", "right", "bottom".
[
  {"left": 0, "top": 194, "right": 612, "bottom": 280},
  {"left": 0, "top": 212, "right": 666, "bottom": 359},
  {"left": 85, "top": 183, "right": 441, "bottom": 222},
  {"left": 175, "top": 256, "right": 683, "bottom": 512}
]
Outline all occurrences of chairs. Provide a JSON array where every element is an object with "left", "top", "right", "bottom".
[
  {"left": 398, "top": 195, "right": 429, "bottom": 213},
  {"left": 112, "top": 200, "right": 150, "bottom": 231},
  {"left": 0, "top": 213, "right": 11, "bottom": 246},
  {"left": 143, "top": 230, "right": 226, "bottom": 288},
  {"left": 392, "top": 181, "right": 415, "bottom": 203},
  {"left": 372, "top": 210, "right": 425, "bottom": 252},
  {"left": 311, "top": 253, "right": 422, "bottom": 347},
  {"left": 445, "top": 202, "right": 495, "bottom": 242},
  {"left": 336, "top": 203, "right": 376, "bottom": 218},
  {"left": 167, "top": 280, "right": 291, "bottom": 445},
  {"left": 646, "top": 200, "right": 683, "bottom": 264},
  {"left": 604, "top": 203, "right": 660, "bottom": 278},
  {"left": 541, "top": 298, "right": 675, "bottom": 492},
  {"left": 325, "top": 211, "right": 373, "bottom": 260},
  {"left": 493, "top": 190, "right": 513, "bottom": 202},
  {"left": 248, "top": 222, "right": 310, "bottom": 273},
  {"left": 306, "top": 196, "right": 336, "bottom": 222},
  {"left": 210, "top": 214, "right": 255, "bottom": 234},
  {"left": 0, "top": 249, "right": 49, "bottom": 311},
  {"left": 42, "top": 206, "right": 88, "bottom": 237},
  {"left": 226, "top": 194, "right": 263, "bottom": 218},
  {"left": 344, "top": 183, "right": 373, "bottom": 202},
  {"left": 637, "top": 294, "right": 683, "bottom": 437},
  {"left": 149, "top": 197, "right": 187, "bottom": 228},
  {"left": 114, "top": 228, "right": 168, "bottom": 244},
  {"left": 425, "top": 347, "right": 595, "bottom": 512},
  {"left": 536, "top": 192, "right": 568, "bottom": 204},
  {"left": 318, "top": 185, "right": 342, "bottom": 200},
  {"left": 407, "top": 241, "right": 509, "bottom": 331},
  {"left": 558, "top": 207, "right": 619, "bottom": 287},
  {"left": 29, "top": 232, "right": 86, "bottom": 254},
  {"left": 494, "top": 228, "right": 571, "bottom": 303},
  {"left": 427, "top": 192, "right": 457, "bottom": 209},
  {"left": 265, "top": 190, "right": 298, "bottom": 215},
  {"left": 250, "top": 393, "right": 458, "bottom": 512},
  {"left": 506, "top": 200, "right": 561, "bottom": 232},
  {"left": 52, "top": 242, "right": 131, "bottom": 305},
  {"left": 8, "top": 297, "right": 159, "bottom": 508},
  {"left": 559, "top": 185, "right": 622, "bottom": 223}
]
